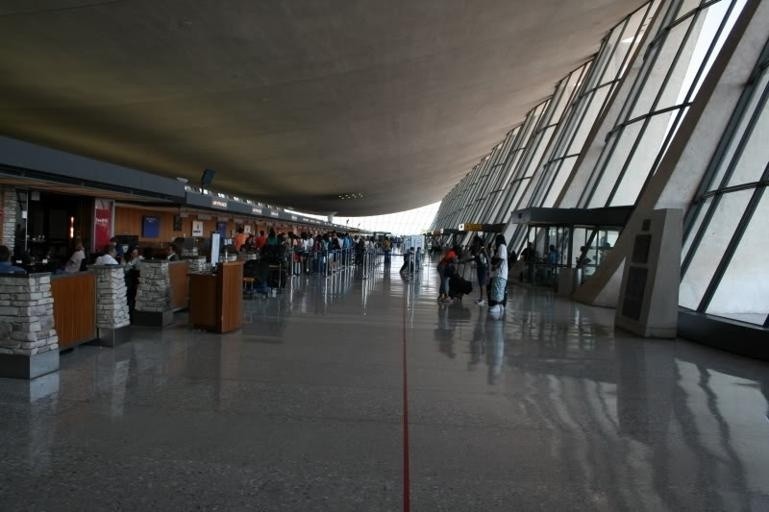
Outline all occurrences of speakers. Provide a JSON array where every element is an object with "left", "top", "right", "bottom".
[{"left": 201, "top": 169, "right": 216, "bottom": 185}]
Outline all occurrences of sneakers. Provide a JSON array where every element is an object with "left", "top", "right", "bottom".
[{"left": 436, "top": 296, "right": 505, "bottom": 313}]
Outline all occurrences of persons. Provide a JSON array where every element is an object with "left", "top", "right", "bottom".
[
  {"left": 52, "top": 233, "right": 179, "bottom": 275},
  {"left": 396, "top": 246, "right": 428, "bottom": 285},
  {"left": 433, "top": 301, "right": 510, "bottom": 388},
  {"left": 288, "top": 231, "right": 392, "bottom": 275},
  {"left": 436, "top": 245, "right": 464, "bottom": 303},
  {"left": 429, "top": 236, "right": 592, "bottom": 289},
  {"left": 233, "top": 227, "right": 292, "bottom": 297},
  {"left": 469, "top": 241, "right": 492, "bottom": 306},
  {"left": 0, "top": 245, "right": 27, "bottom": 274},
  {"left": 486, "top": 234, "right": 509, "bottom": 313}
]
